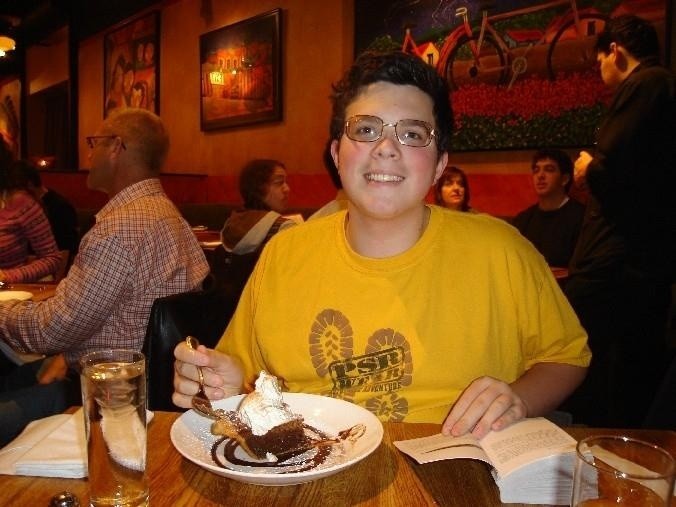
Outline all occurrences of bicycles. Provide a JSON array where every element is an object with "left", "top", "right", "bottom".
[{"left": 443, "top": 0, "right": 614, "bottom": 96}]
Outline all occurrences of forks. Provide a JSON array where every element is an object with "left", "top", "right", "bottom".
[{"left": 182, "top": 336, "right": 219, "bottom": 422}]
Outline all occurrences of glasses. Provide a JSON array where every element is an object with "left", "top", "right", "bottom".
[
  {"left": 342, "top": 114, "right": 436, "bottom": 148},
  {"left": 86, "top": 135, "right": 127, "bottom": 151}
]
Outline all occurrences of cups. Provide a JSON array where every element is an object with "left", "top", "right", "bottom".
[
  {"left": 569, "top": 433, "right": 676, "bottom": 506},
  {"left": 77, "top": 349, "right": 149, "bottom": 506}
]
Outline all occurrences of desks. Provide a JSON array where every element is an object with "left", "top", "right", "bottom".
[
  {"left": 1, "top": 401, "right": 673, "bottom": 504},
  {"left": 1, "top": 169, "right": 674, "bottom": 387}
]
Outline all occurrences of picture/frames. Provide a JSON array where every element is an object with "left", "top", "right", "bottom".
[
  {"left": 102, "top": 12, "right": 163, "bottom": 123},
  {"left": 339, "top": 0, "right": 676, "bottom": 166},
  {"left": 198, "top": 6, "right": 285, "bottom": 131}
]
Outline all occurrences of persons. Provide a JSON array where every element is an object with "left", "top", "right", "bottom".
[
  {"left": 509, "top": 150, "right": 582, "bottom": 265},
  {"left": 303, "top": 141, "right": 348, "bottom": 219},
  {"left": 165, "top": 46, "right": 598, "bottom": 437},
  {"left": 433, "top": 168, "right": 480, "bottom": 213},
  {"left": 570, "top": 8, "right": 675, "bottom": 428},
  {"left": 14, "top": 158, "right": 78, "bottom": 253},
  {"left": 219, "top": 157, "right": 302, "bottom": 250},
  {"left": 0, "top": 132, "right": 60, "bottom": 286},
  {"left": 0, "top": 106, "right": 216, "bottom": 443}
]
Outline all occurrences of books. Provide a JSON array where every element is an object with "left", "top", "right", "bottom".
[{"left": 389, "top": 409, "right": 600, "bottom": 505}]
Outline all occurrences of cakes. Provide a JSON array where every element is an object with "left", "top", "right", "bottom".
[{"left": 209, "top": 372, "right": 310, "bottom": 460}]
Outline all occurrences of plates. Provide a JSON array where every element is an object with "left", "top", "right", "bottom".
[
  {"left": 166, "top": 391, "right": 385, "bottom": 486},
  {"left": 0, "top": 290, "right": 34, "bottom": 302}
]
